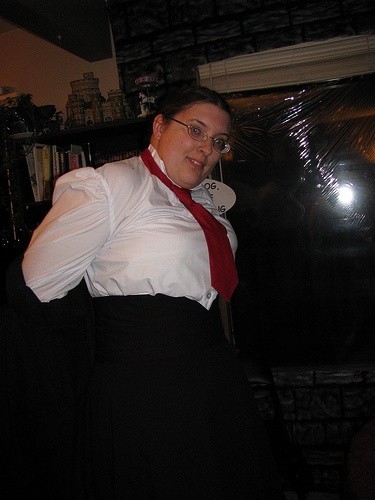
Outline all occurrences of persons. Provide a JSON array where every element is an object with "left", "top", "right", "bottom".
[{"left": 20, "top": 86, "right": 238, "bottom": 500}]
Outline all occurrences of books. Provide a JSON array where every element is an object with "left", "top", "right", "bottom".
[{"left": 23, "top": 141, "right": 91, "bottom": 203}]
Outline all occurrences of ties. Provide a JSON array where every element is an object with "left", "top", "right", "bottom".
[{"left": 139, "top": 148, "right": 239, "bottom": 303}]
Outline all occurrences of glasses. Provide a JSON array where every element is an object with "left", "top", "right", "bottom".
[{"left": 169, "top": 117, "right": 231, "bottom": 154}]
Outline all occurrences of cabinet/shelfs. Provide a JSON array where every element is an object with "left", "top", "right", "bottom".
[{"left": 0, "top": 115, "right": 155, "bottom": 239}]
